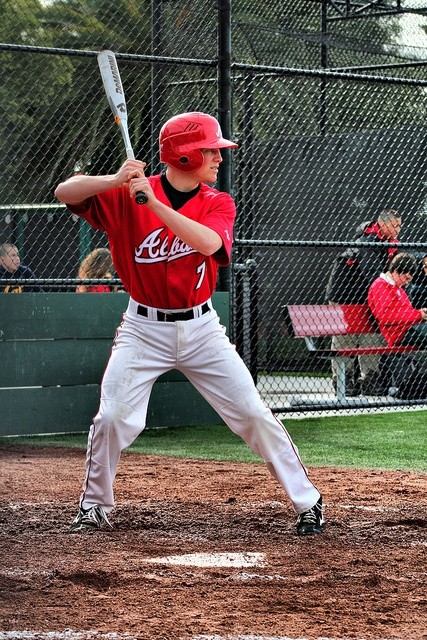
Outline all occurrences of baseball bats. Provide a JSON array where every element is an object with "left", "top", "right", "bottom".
[{"left": 96, "top": 49, "right": 148, "bottom": 204}]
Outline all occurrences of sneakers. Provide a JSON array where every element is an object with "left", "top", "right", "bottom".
[
  {"left": 69, "top": 507, "right": 115, "bottom": 535},
  {"left": 361, "top": 383, "right": 386, "bottom": 396},
  {"left": 298, "top": 496, "right": 326, "bottom": 537},
  {"left": 334, "top": 385, "right": 356, "bottom": 396}
]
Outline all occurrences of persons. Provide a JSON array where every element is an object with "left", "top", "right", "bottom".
[
  {"left": 367, "top": 252, "right": 426, "bottom": 399},
  {"left": 75, "top": 248, "right": 117, "bottom": 292},
  {"left": 402, "top": 252, "right": 426, "bottom": 310},
  {"left": 0, "top": 243, "right": 44, "bottom": 293},
  {"left": 324, "top": 209, "right": 401, "bottom": 397},
  {"left": 54, "top": 111, "right": 323, "bottom": 536}
]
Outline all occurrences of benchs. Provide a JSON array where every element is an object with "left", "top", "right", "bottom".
[{"left": 279, "top": 303, "right": 425, "bottom": 407}]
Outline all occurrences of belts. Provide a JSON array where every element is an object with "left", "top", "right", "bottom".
[{"left": 137, "top": 305, "right": 212, "bottom": 323}]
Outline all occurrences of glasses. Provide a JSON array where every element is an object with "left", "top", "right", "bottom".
[{"left": 392, "top": 223, "right": 404, "bottom": 229}]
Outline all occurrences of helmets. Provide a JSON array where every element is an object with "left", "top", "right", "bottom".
[{"left": 160, "top": 112, "right": 239, "bottom": 175}]
